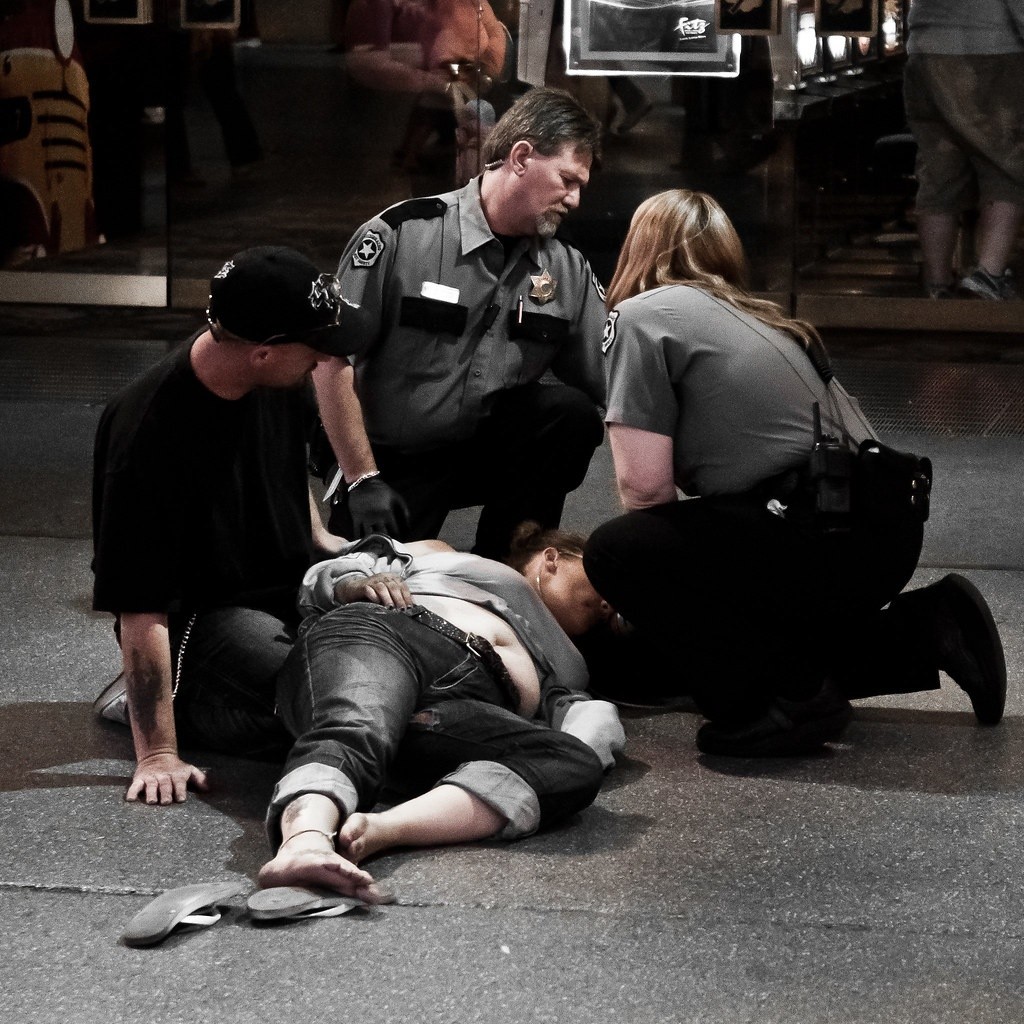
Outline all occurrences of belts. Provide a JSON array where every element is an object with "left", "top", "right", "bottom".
[{"left": 388, "top": 603, "right": 520, "bottom": 716}]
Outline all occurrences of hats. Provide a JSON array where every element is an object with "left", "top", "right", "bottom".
[{"left": 211, "top": 245, "right": 365, "bottom": 357}]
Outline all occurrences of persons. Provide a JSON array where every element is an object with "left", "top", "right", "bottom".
[
  {"left": 345, "top": 0, "right": 516, "bottom": 217},
  {"left": 255, "top": 527, "right": 624, "bottom": 904},
  {"left": 586, "top": 189, "right": 1006, "bottom": 761},
  {"left": 903, "top": 0, "right": 1024, "bottom": 301},
  {"left": 311, "top": 81, "right": 619, "bottom": 572},
  {"left": 87, "top": 244, "right": 368, "bottom": 804}
]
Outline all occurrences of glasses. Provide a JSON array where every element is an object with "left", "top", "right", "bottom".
[
  {"left": 205, "top": 294, "right": 262, "bottom": 346},
  {"left": 559, "top": 549, "right": 637, "bottom": 640}
]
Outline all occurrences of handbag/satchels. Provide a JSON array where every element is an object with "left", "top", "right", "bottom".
[{"left": 450, "top": 81, "right": 497, "bottom": 189}]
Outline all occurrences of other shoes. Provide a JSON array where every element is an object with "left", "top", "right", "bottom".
[
  {"left": 926, "top": 572, "right": 1008, "bottom": 726},
  {"left": 696, "top": 681, "right": 857, "bottom": 757}
]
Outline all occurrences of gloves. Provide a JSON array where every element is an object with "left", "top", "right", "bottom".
[{"left": 349, "top": 474, "right": 414, "bottom": 542}]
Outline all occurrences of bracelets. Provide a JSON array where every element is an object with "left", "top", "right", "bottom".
[{"left": 348, "top": 470, "right": 379, "bottom": 491}]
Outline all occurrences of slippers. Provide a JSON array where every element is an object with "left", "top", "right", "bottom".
[
  {"left": 125, "top": 881, "right": 242, "bottom": 947},
  {"left": 247, "top": 883, "right": 396, "bottom": 920}
]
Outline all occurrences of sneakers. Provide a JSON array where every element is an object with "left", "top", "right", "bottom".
[
  {"left": 961, "top": 266, "right": 1023, "bottom": 303},
  {"left": 92, "top": 671, "right": 132, "bottom": 726},
  {"left": 924, "top": 271, "right": 966, "bottom": 299}
]
